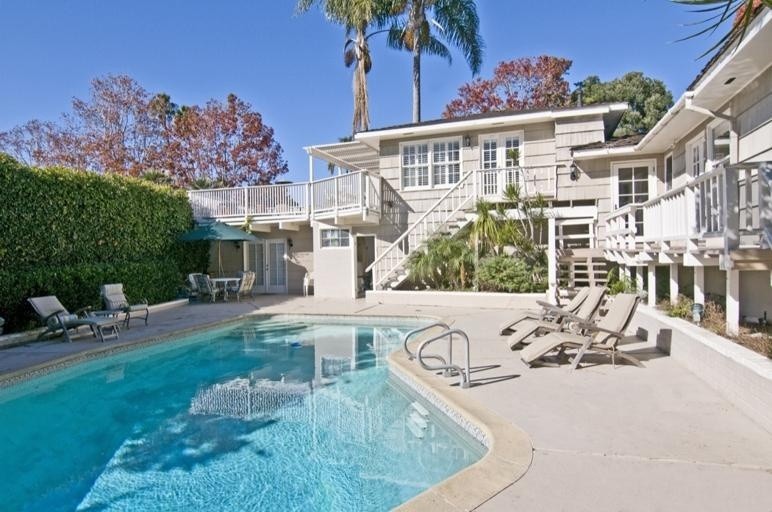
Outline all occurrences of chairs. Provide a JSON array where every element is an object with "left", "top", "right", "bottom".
[
  {"left": 501, "top": 285, "right": 644, "bottom": 373},
  {"left": 189, "top": 271, "right": 254, "bottom": 303},
  {"left": 28, "top": 283, "right": 150, "bottom": 344}
]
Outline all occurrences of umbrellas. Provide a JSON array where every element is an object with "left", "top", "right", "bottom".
[{"left": 179, "top": 221, "right": 260, "bottom": 291}]
